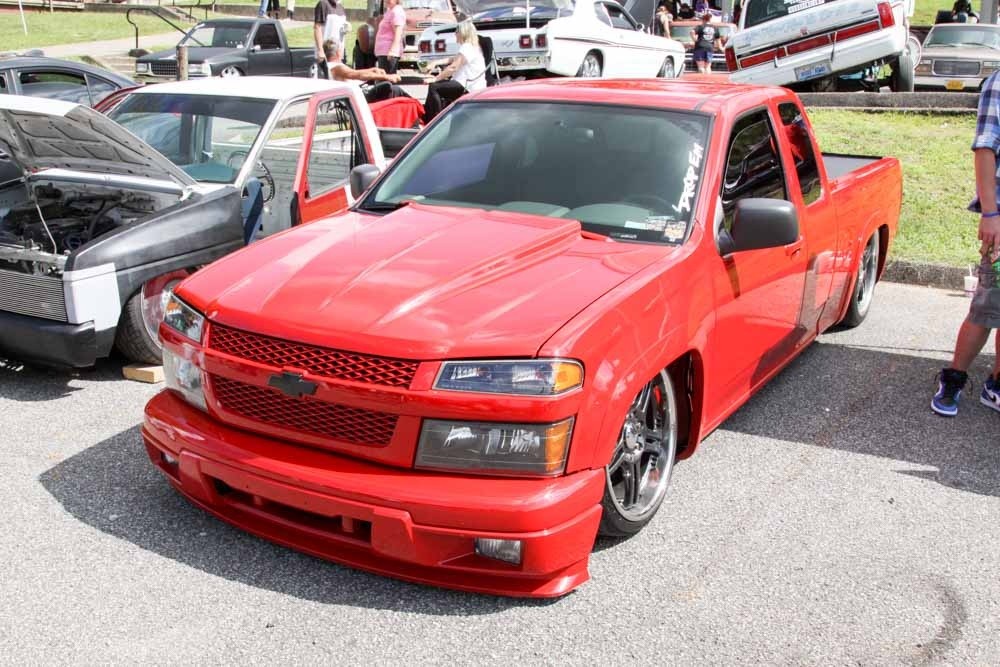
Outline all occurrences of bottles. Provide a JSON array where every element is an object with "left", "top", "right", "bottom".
[{"left": 963, "top": 263, "right": 979, "bottom": 296}]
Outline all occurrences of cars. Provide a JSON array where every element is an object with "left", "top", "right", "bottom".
[
  {"left": 350, "top": 0, "right": 738, "bottom": 76},
  {"left": 0, "top": 54, "right": 142, "bottom": 188},
  {"left": 722, "top": 0, "right": 917, "bottom": 94},
  {"left": 910, "top": 22, "right": 1000, "bottom": 91}
]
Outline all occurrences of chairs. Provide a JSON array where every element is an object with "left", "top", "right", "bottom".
[{"left": 318, "top": 58, "right": 468, "bottom": 128}]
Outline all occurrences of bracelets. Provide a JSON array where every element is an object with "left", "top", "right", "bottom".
[{"left": 981, "top": 212, "right": 1000, "bottom": 217}]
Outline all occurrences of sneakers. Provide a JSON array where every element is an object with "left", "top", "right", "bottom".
[
  {"left": 930, "top": 368, "right": 968, "bottom": 416},
  {"left": 979, "top": 374, "right": 1000, "bottom": 413}
]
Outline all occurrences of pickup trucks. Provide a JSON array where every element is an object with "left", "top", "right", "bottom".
[
  {"left": 0, "top": 76, "right": 423, "bottom": 381},
  {"left": 138, "top": 74, "right": 905, "bottom": 602},
  {"left": 133, "top": 16, "right": 347, "bottom": 80}
]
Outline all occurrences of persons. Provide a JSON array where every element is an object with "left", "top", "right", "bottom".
[
  {"left": 929, "top": 69, "right": 1000, "bottom": 417},
  {"left": 420, "top": 21, "right": 487, "bottom": 126},
  {"left": 314, "top": 0, "right": 351, "bottom": 78},
  {"left": 323, "top": 40, "right": 421, "bottom": 128},
  {"left": 258, "top": 0, "right": 295, "bottom": 20},
  {"left": 690, "top": 14, "right": 723, "bottom": 72},
  {"left": 649, "top": 0, "right": 723, "bottom": 40},
  {"left": 951, "top": 0, "right": 971, "bottom": 23},
  {"left": 352, "top": 0, "right": 406, "bottom": 74}
]
